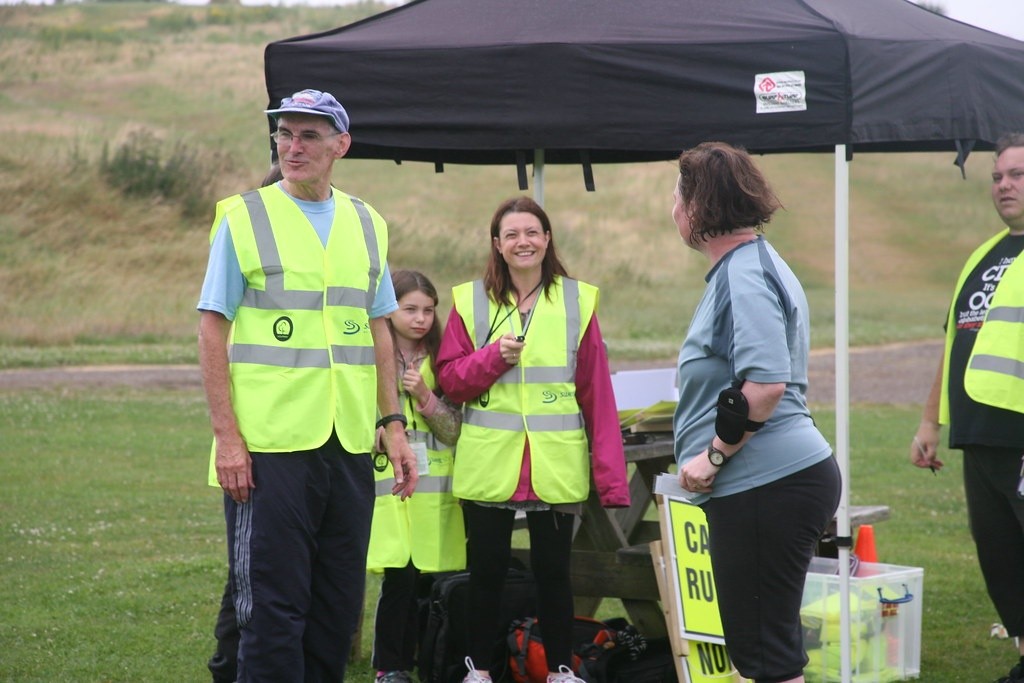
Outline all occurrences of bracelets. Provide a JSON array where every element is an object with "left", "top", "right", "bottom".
[{"left": 375, "top": 414, "right": 408, "bottom": 430}]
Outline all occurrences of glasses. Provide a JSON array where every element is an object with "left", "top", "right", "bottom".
[{"left": 270, "top": 130, "right": 341, "bottom": 144}]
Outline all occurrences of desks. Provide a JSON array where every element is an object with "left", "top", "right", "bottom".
[{"left": 507, "top": 437, "right": 855, "bottom": 683}]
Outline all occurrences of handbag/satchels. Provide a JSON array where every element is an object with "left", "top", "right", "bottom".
[
  {"left": 507, "top": 613, "right": 614, "bottom": 683},
  {"left": 420, "top": 555, "right": 543, "bottom": 683},
  {"left": 577, "top": 636, "right": 678, "bottom": 683}
]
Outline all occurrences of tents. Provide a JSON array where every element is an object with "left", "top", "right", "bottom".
[{"left": 263, "top": 1, "right": 1024, "bottom": 683}]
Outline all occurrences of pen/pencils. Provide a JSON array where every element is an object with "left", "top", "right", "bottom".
[{"left": 914, "top": 436, "right": 938, "bottom": 476}]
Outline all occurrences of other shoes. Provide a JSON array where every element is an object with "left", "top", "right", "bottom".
[
  {"left": 992, "top": 656, "right": 1024, "bottom": 683},
  {"left": 462, "top": 654, "right": 493, "bottom": 683},
  {"left": 374, "top": 670, "right": 411, "bottom": 683},
  {"left": 546, "top": 665, "right": 584, "bottom": 683}
]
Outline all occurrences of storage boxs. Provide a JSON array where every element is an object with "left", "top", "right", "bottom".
[{"left": 800, "top": 556, "right": 924, "bottom": 683}]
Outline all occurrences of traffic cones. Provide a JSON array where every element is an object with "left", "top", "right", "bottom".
[{"left": 855, "top": 522, "right": 878, "bottom": 577}]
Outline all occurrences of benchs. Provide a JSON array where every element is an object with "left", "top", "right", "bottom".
[{"left": 617, "top": 504, "right": 892, "bottom": 564}]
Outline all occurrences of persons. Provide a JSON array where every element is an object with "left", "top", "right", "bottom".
[
  {"left": 366, "top": 270, "right": 468, "bottom": 683},
  {"left": 910, "top": 144, "right": 1024, "bottom": 682},
  {"left": 433, "top": 198, "right": 632, "bottom": 683},
  {"left": 198, "top": 88, "right": 418, "bottom": 683},
  {"left": 671, "top": 141, "right": 842, "bottom": 683}
]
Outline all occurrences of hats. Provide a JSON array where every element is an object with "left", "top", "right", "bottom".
[{"left": 264, "top": 88, "right": 350, "bottom": 133}]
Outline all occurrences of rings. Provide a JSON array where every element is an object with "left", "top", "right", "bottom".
[
  {"left": 514, "top": 354, "right": 515, "bottom": 359},
  {"left": 692, "top": 483, "right": 699, "bottom": 489}
]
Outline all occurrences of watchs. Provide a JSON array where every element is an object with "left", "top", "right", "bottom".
[{"left": 708, "top": 438, "right": 733, "bottom": 467}]
